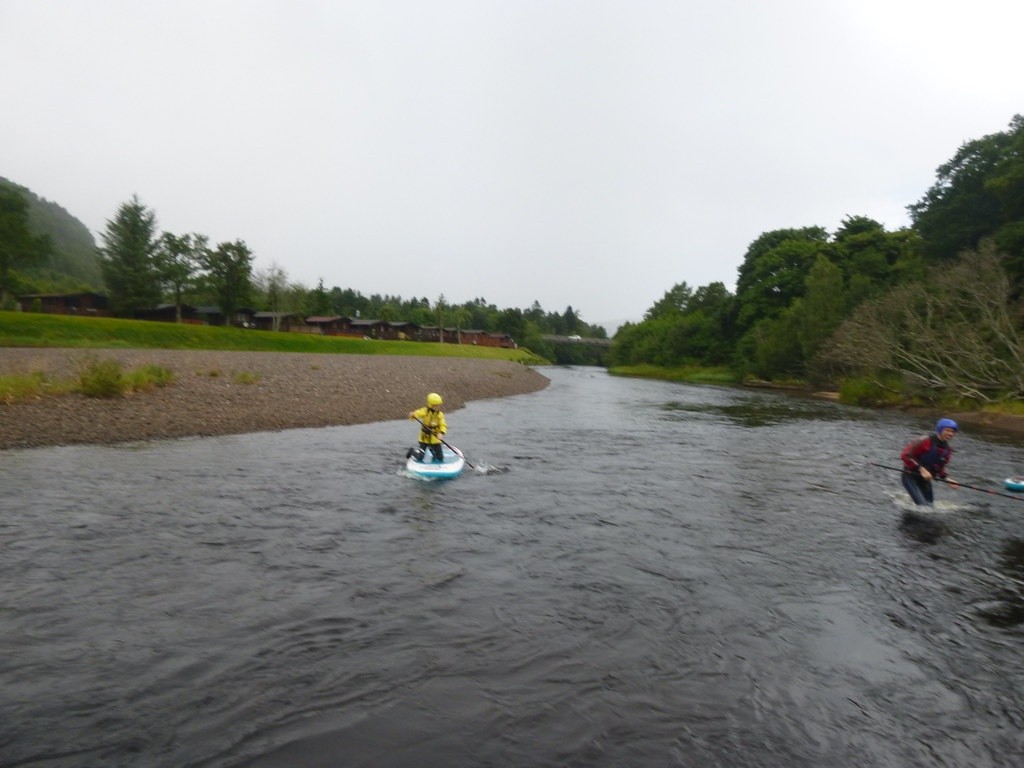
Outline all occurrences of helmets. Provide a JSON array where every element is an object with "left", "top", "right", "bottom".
[
  {"left": 427, "top": 393, "right": 443, "bottom": 409},
  {"left": 936, "top": 418, "right": 958, "bottom": 433}
]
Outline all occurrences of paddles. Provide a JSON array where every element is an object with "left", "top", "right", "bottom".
[
  {"left": 1002, "top": 476, "right": 1023, "bottom": 493},
  {"left": 411, "top": 414, "right": 486, "bottom": 476},
  {"left": 867, "top": 459, "right": 1024, "bottom": 504}
]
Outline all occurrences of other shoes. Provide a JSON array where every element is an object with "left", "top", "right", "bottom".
[{"left": 406, "top": 447, "right": 414, "bottom": 459}]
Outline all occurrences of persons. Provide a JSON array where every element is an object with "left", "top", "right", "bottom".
[
  {"left": 404, "top": 393, "right": 447, "bottom": 461},
  {"left": 899, "top": 417, "right": 961, "bottom": 506}
]
